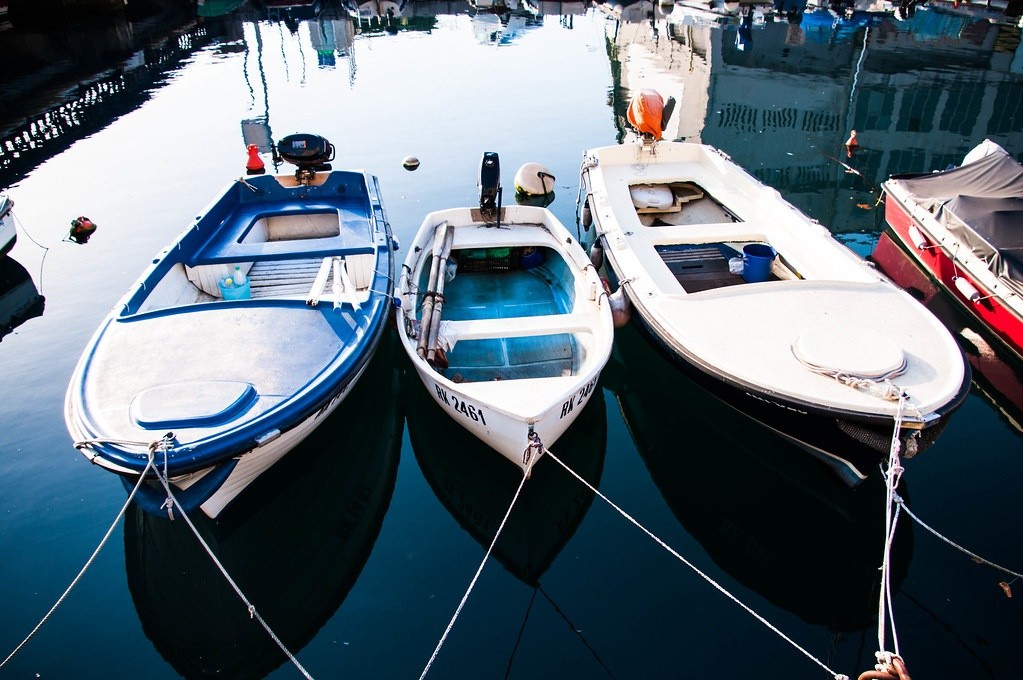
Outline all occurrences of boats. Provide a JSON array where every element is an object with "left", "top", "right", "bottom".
[
  {"left": 864, "top": 224, "right": 1023, "bottom": 435},
  {"left": 121, "top": 298, "right": 406, "bottom": 679},
  {"left": 572, "top": 139, "right": 976, "bottom": 487},
  {"left": 862, "top": 137, "right": 1023, "bottom": 371},
  {"left": 394, "top": 146, "right": 617, "bottom": 478},
  {"left": 61, "top": 133, "right": 400, "bottom": 516},
  {"left": 397, "top": 344, "right": 608, "bottom": 589},
  {"left": 588, "top": 253, "right": 918, "bottom": 639},
  {"left": 0, "top": 190, "right": 18, "bottom": 258},
  {"left": 0, "top": 252, "right": 46, "bottom": 344}
]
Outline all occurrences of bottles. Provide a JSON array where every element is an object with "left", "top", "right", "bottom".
[
  {"left": 225, "top": 277, "right": 235, "bottom": 289},
  {"left": 220, "top": 279, "right": 226, "bottom": 287},
  {"left": 234, "top": 266, "right": 247, "bottom": 287}
]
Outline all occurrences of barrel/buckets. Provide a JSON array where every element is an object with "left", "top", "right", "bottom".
[
  {"left": 218, "top": 275, "right": 250, "bottom": 300},
  {"left": 742, "top": 243, "right": 777, "bottom": 283}
]
